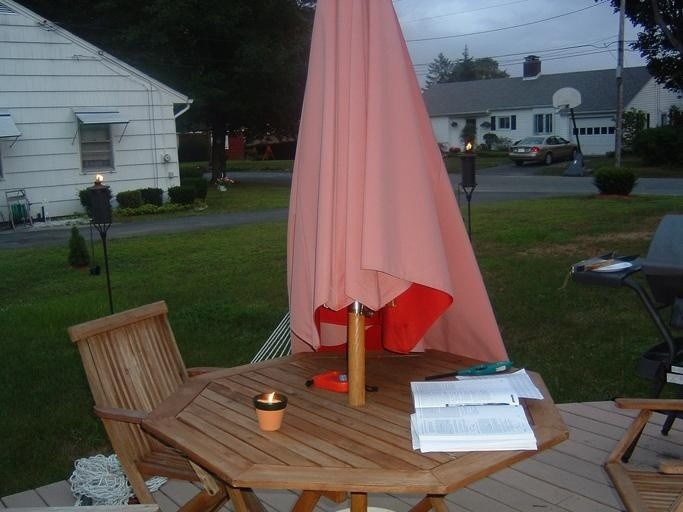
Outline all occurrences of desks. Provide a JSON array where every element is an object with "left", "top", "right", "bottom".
[{"left": 141, "top": 347, "right": 569, "bottom": 512}]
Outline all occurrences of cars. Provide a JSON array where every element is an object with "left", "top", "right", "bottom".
[{"left": 508, "top": 135, "right": 579, "bottom": 166}]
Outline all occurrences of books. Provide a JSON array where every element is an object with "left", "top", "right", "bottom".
[{"left": 409, "top": 377, "right": 539, "bottom": 453}]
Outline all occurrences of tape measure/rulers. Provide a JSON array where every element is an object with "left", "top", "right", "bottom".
[{"left": 305, "top": 369, "right": 348, "bottom": 393}]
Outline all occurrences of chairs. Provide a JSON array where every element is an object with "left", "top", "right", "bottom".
[
  {"left": 604, "top": 398, "right": 683, "bottom": 512},
  {"left": 67, "top": 300, "right": 230, "bottom": 511},
  {"left": 570, "top": 212, "right": 683, "bottom": 463}
]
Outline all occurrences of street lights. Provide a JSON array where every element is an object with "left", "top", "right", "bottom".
[
  {"left": 75, "top": 176, "right": 114, "bottom": 317},
  {"left": 458, "top": 140, "right": 477, "bottom": 243}
]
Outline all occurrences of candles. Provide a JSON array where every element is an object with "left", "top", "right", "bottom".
[
  {"left": 89, "top": 173, "right": 112, "bottom": 223},
  {"left": 462, "top": 141, "right": 476, "bottom": 185},
  {"left": 253, "top": 392, "right": 287, "bottom": 431}
]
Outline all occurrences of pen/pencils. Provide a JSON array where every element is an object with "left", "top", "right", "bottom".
[{"left": 445, "top": 401, "right": 512, "bottom": 407}]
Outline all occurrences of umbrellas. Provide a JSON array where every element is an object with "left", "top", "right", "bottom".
[{"left": 286, "top": 0, "right": 512, "bottom": 512}]
